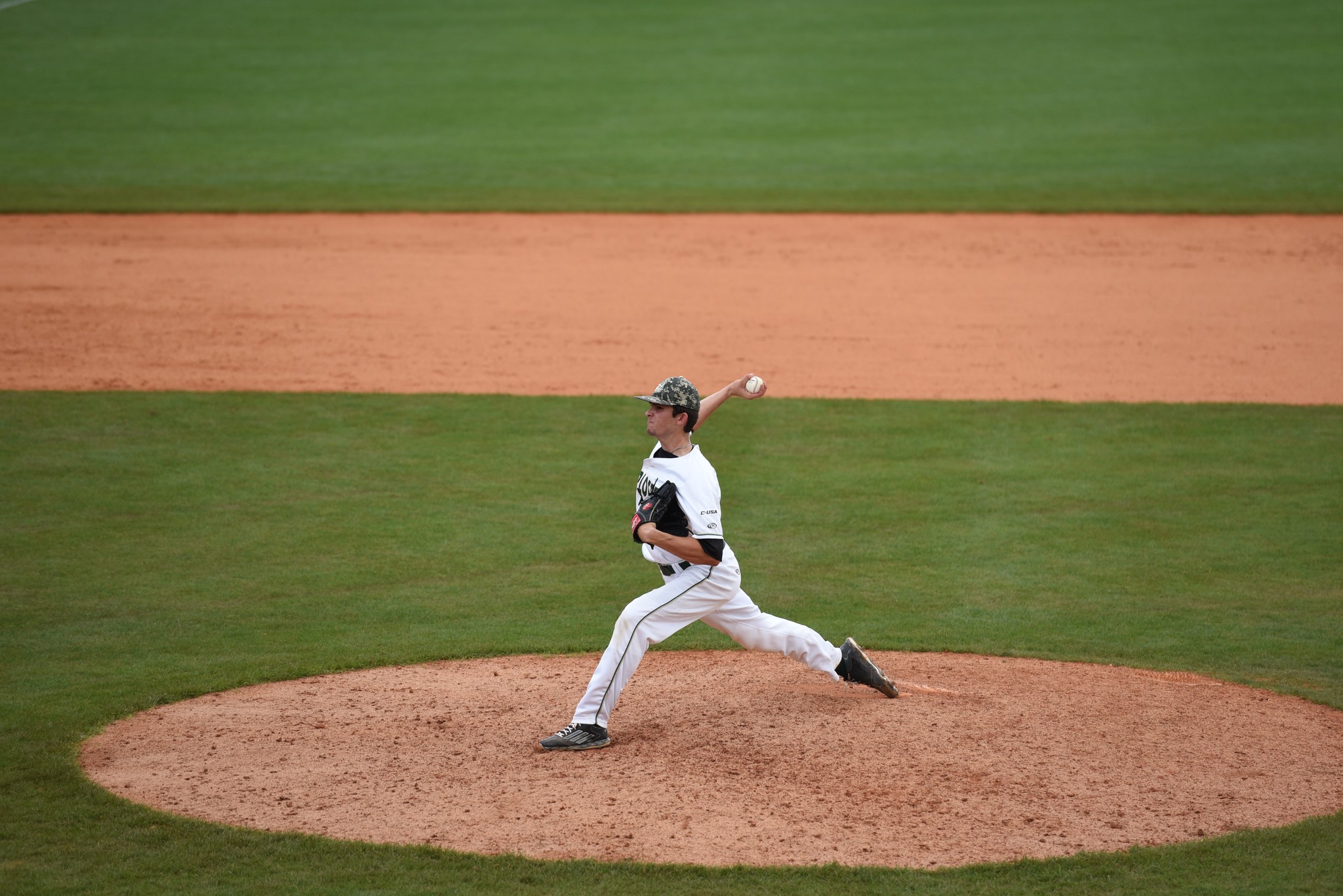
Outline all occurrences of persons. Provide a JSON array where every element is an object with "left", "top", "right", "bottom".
[{"left": 536, "top": 373, "right": 899, "bottom": 752}]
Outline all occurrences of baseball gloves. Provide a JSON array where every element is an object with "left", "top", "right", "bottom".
[{"left": 631, "top": 480, "right": 690, "bottom": 544}]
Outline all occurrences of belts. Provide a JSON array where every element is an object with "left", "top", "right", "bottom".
[{"left": 658, "top": 561, "right": 690, "bottom": 576}]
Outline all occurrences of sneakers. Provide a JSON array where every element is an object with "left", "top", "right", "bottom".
[
  {"left": 539, "top": 722, "right": 609, "bottom": 751},
  {"left": 838, "top": 637, "right": 899, "bottom": 699}
]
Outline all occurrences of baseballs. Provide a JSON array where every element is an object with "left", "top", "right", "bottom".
[{"left": 745, "top": 376, "right": 763, "bottom": 393}]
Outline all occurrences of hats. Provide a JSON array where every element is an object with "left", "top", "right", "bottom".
[{"left": 634, "top": 376, "right": 700, "bottom": 412}]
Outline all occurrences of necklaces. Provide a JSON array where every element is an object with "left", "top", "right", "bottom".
[{"left": 671, "top": 442, "right": 691, "bottom": 454}]
